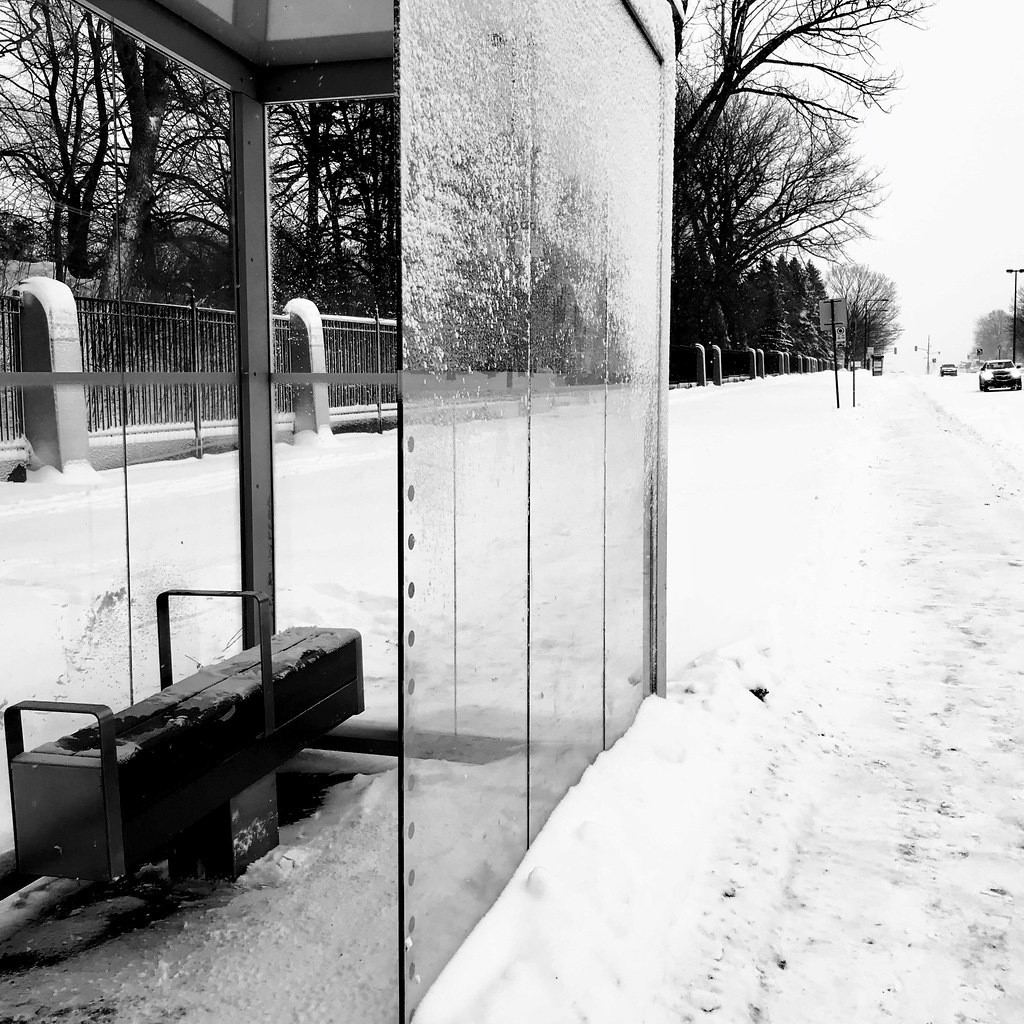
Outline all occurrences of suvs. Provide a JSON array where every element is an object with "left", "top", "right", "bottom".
[
  {"left": 978, "top": 359, "right": 1023, "bottom": 392},
  {"left": 939, "top": 364, "right": 959, "bottom": 377}
]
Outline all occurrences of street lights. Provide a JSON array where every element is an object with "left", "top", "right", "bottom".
[
  {"left": 1006, "top": 269, "right": 1024, "bottom": 365},
  {"left": 864, "top": 298, "right": 889, "bottom": 369},
  {"left": 864, "top": 309, "right": 889, "bottom": 369}
]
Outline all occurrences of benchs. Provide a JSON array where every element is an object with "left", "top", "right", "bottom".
[{"left": 0, "top": 588, "right": 369, "bottom": 888}]
